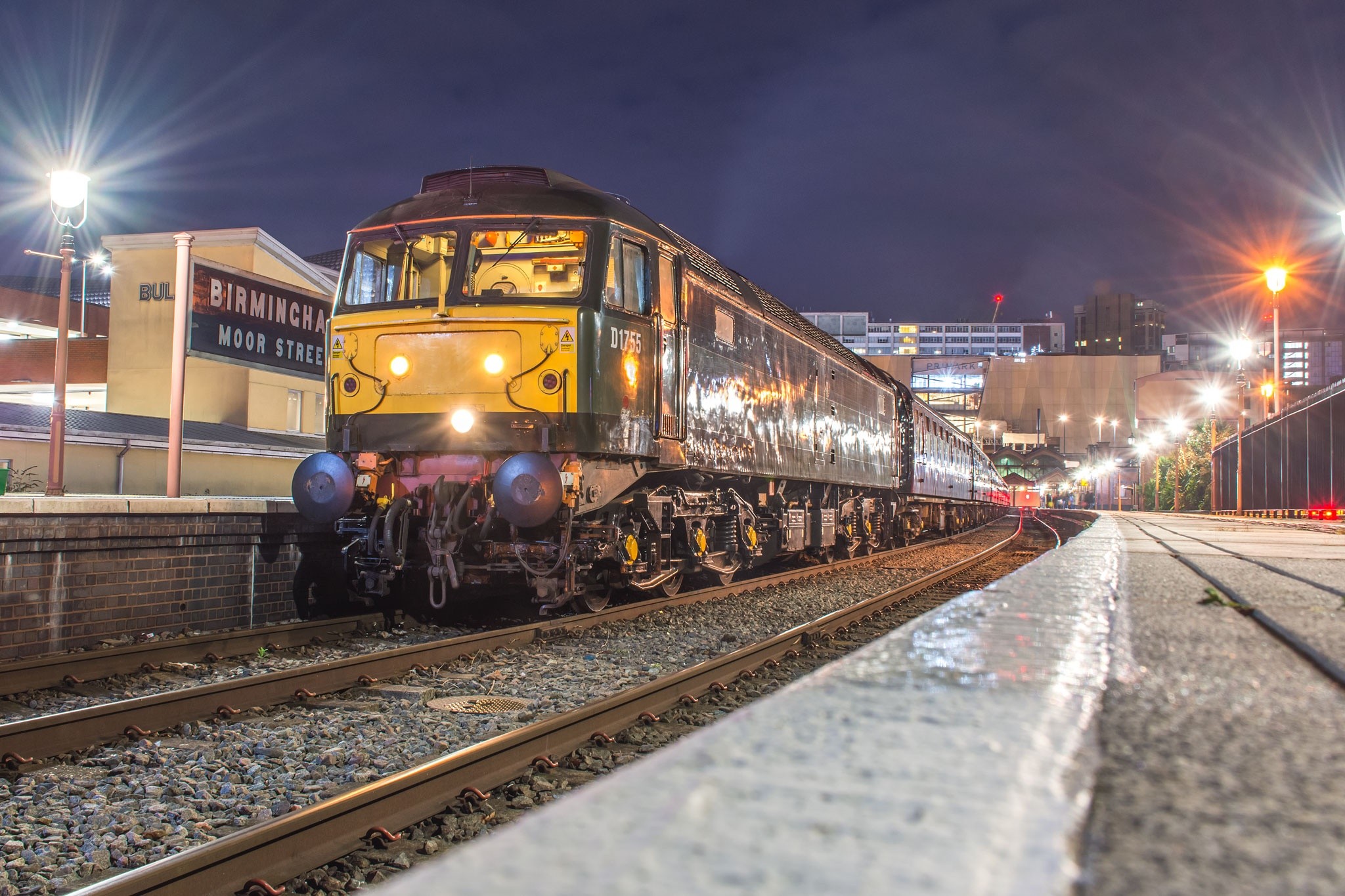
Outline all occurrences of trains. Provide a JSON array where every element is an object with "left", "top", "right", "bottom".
[{"left": 292, "top": 163, "right": 1010, "bottom": 616}]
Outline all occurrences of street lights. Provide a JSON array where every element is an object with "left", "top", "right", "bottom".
[
  {"left": 45, "top": 168, "right": 93, "bottom": 497},
  {"left": 1150, "top": 431, "right": 1163, "bottom": 512},
  {"left": 1111, "top": 420, "right": 1118, "bottom": 447},
  {"left": 1169, "top": 417, "right": 1184, "bottom": 513},
  {"left": 1265, "top": 266, "right": 1287, "bottom": 417},
  {"left": 1203, "top": 388, "right": 1219, "bottom": 511},
  {"left": 1229, "top": 338, "right": 1252, "bottom": 518},
  {"left": 1060, "top": 414, "right": 1067, "bottom": 454},
  {"left": 1115, "top": 458, "right": 1123, "bottom": 511},
  {"left": 1257, "top": 341, "right": 1274, "bottom": 422},
  {"left": 1096, "top": 417, "right": 1103, "bottom": 442}
]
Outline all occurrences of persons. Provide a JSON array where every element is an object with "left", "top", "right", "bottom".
[
  {"left": 1040, "top": 491, "right": 1074, "bottom": 509},
  {"left": 1078, "top": 490, "right": 1095, "bottom": 510}
]
